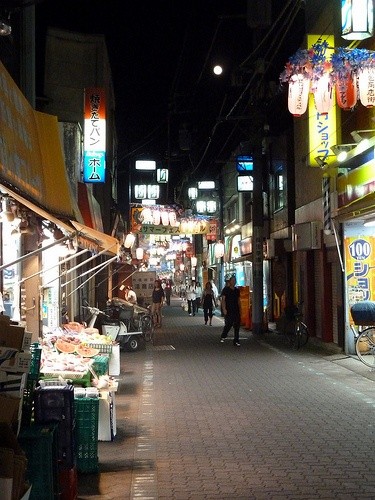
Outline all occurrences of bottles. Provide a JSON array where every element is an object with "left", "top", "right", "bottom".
[{"left": 59, "top": 461, "right": 79, "bottom": 500}]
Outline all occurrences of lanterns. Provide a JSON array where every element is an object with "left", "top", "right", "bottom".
[
  {"left": 315, "top": 71, "right": 331, "bottom": 115},
  {"left": 287, "top": 72, "right": 311, "bottom": 118},
  {"left": 336, "top": 61, "right": 358, "bottom": 112},
  {"left": 356, "top": 62, "right": 375, "bottom": 109},
  {"left": 123, "top": 204, "right": 225, "bottom": 278}
]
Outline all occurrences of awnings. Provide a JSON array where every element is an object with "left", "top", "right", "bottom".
[{"left": 1, "top": 178, "right": 148, "bottom": 300}]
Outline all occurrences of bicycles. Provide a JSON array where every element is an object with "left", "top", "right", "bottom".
[
  {"left": 117, "top": 305, "right": 154, "bottom": 342},
  {"left": 283, "top": 299, "right": 310, "bottom": 352},
  {"left": 354, "top": 325, "right": 375, "bottom": 370}
]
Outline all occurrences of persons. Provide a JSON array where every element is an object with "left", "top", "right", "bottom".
[
  {"left": 202, "top": 282, "right": 218, "bottom": 326},
  {"left": 152, "top": 279, "right": 165, "bottom": 328},
  {"left": 186, "top": 280, "right": 197, "bottom": 316},
  {"left": 124, "top": 286, "right": 138, "bottom": 305},
  {"left": 163, "top": 283, "right": 171, "bottom": 306},
  {"left": 194, "top": 282, "right": 202, "bottom": 313},
  {"left": 218, "top": 275, "right": 241, "bottom": 347}
]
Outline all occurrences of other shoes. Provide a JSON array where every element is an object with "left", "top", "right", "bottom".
[
  {"left": 221, "top": 338, "right": 225, "bottom": 342},
  {"left": 193, "top": 314, "right": 195, "bottom": 316},
  {"left": 189, "top": 313, "right": 191, "bottom": 316},
  {"left": 209, "top": 323, "right": 212, "bottom": 326},
  {"left": 205, "top": 322, "right": 207, "bottom": 325},
  {"left": 235, "top": 342, "right": 240, "bottom": 345}
]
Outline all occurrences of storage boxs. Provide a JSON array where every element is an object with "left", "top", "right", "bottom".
[{"left": 0, "top": 310, "right": 120, "bottom": 500}]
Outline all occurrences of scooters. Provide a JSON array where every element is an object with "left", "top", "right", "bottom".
[{"left": 81, "top": 298, "right": 143, "bottom": 353}]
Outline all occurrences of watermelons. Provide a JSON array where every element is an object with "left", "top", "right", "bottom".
[
  {"left": 56, "top": 343, "right": 78, "bottom": 353},
  {"left": 76, "top": 348, "right": 100, "bottom": 357}
]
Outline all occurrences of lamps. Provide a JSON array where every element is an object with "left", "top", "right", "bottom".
[
  {"left": 186, "top": 185, "right": 200, "bottom": 201},
  {"left": 212, "top": 240, "right": 225, "bottom": 259},
  {"left": 330, "top": 142, "right": 358, "bottom": 162},
  {"left": 123, "top": 232, "right": 137, "bottom": 248},
  {"left": 148, "top": 184, "right": 160, "bottom": 199},
  {"left": 133, "top": 184, "right": 147, "bottom": 199},
  {"left": 340, "top": 0, "right": 375, "bottom": 40},
  {"left": 350, "top": 129, "right": 375, "bottom": 151},
  {"left": 157, "top": 167, "right": 168, "bottom": 184},
  {"left": 313, "top": 154, "right": 336, "bottom": 170}
]
